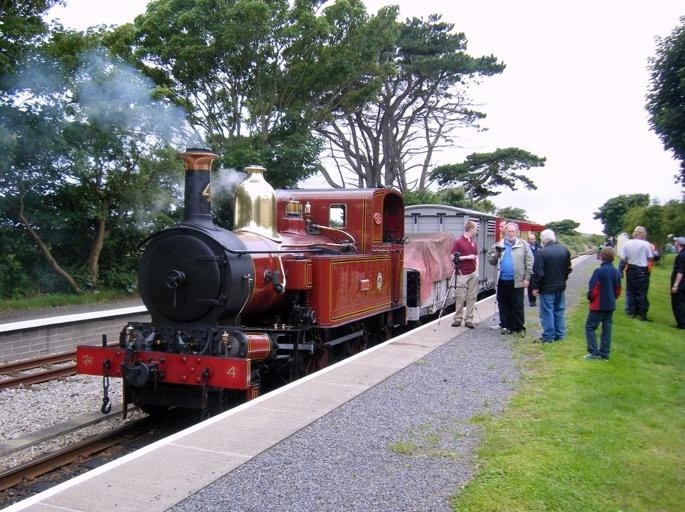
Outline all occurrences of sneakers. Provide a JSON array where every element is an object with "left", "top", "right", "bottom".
[
  {"left": 529, "top": 302, "right": 535, "bottom": 306},
  {"left": 579, "top": 353, "right": 609, "bottom": 361},
  {"left": 533, "top": 335, "right": 564, "bottom": 344},
  {"left": 489, "top": 324, "right": 527, "bottom": 338}
]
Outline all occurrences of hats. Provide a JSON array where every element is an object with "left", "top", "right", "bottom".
[{"left": 673, "top": 236, "right": 685, "bottom": 244}]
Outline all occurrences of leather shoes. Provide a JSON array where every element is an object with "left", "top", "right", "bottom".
[
  {"left": 629, "top": 313, "right": 636, "bottom": 319},
  {"left": 465, "top": 322, "right": 474, "bottom": 329},
  {"left": 638, "top": 316, "right": 653, "bottom": 323},
  {"left": 451, "top": 320, "right": 460, "bottom": 327}
]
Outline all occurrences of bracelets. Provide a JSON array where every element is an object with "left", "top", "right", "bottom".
[{"left": 673, "top": 285, "right": 679, "bottom": 288}]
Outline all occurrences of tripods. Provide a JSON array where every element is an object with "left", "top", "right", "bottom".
[{"left": 434, "top": 270, "right": 483, "bottom": 329}]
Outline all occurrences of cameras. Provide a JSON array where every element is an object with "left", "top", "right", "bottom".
[{"left": 451, "top": 251, "right": 461, "bottom": 264}]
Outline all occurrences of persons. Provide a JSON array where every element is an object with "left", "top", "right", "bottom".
[
  {"left": 669, "top": 237, "right": 685, "bottom": 329},
  {"left": 531, "top": 229, "right": 573, "bottom": 344},
  {"left": 616, "top": 236, "right": 660, "bottom": 316},
  {"left": 451, "top": 220, "right": 480, "bottom": 329},
  {"left": 489, "top": 218, "right": 512, "bottom": 331},
  {"left": 527, "top": 232, "right": 541, "bottom": 306},
  {"left": 487, "top": 222, "right": 536, "bottom": 337},
  {"left": 623, "top": 224, "right": 654, "bottom": 322},
  {"left": 580, "top": 246, "right": 623, "bottom": 362}
]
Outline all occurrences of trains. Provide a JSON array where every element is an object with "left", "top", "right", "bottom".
[{"left": 75, "top": 147, "right": 542, "bottom": 429}]
